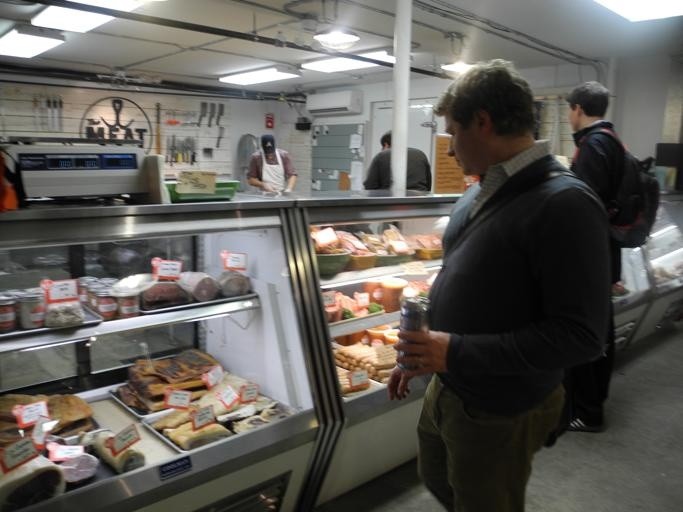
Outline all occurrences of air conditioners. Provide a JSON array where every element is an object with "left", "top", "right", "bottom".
[{"left": 305, "top": 89, "right": 364, "bottom": 117}]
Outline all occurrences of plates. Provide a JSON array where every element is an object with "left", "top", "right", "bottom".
[
  {"left": 108, "top": 272, "right": 156, "bottom": 298},
  {"left": 415, "top": 248, "right": 443, "bottom": 260}
]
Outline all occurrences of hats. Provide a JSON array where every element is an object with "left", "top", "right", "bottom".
[{"left": 261, "top": 135, "right": 274, "bottom": 147}]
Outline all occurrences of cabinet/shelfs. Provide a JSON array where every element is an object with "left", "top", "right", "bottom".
[
  {"left": 307, "top": 197, "right": 648, "bottom": 512},
  {"left": 626, "top": 194, "right": 682, "bottom": 350},
  {"left": 0, "top": 209, "right": 334, "bottom": 511}
]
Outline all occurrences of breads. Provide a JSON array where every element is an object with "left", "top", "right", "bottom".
[{"left": 334, "top": 342, "right": 398, "bottom": 394}]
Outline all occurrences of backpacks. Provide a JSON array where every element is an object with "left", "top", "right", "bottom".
[{"left": 571, "top": 127, "right": 658, "bottom": 247}]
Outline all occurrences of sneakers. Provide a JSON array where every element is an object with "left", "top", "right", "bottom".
[{"left": 565, "top": 417, "right": 600, "bottom": 432}]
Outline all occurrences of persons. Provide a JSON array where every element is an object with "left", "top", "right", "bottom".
[
  {"left": 246, "top": 134, "right": 298, "bottom": 197},
  {"left": 363, "top": 130, "right": 433, "bottom": 192},
  {"left": 565, "top": 80, "right": 633, "bottom": 435},
  {"left": 387, "top": 59, "right": 612, "bottom": 512}
]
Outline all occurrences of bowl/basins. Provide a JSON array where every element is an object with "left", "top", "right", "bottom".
[{"left": 316, "top": 249, "right": 350, "bottom": 277}]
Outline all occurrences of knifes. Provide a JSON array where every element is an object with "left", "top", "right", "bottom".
[
  {"left": 32, "top": 97, "right": 64, "bottom": 132},
  {"left": 207, "top": 103, "right": 216, "bottom": 128},
  {"left": 215, "top": 103, "right": 224, "bottom": 126},
  {"left": 216, "top": 126, "right": 225, "bottom": 148},
  {"left": 196, "top": 101, "right": 207, "bottom": 127}
]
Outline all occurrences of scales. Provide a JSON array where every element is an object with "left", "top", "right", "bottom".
[{"left": 0, "top": 135, "right": 152, "bottom": 199}]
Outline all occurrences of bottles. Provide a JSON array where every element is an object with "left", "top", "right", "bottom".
[{"left": 653, "top": 161, "right": 678, "bottom": 193}]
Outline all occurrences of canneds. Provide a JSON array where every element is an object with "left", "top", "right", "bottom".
[
  {"left": 0, "top": 287, "right": 47, "bottom": 331},
  {"left": 398, "top": 296, "right": 432, "bottom": 371},
  {"left": 78, "top": 275, "right": 140, "bottom": 319}
]
